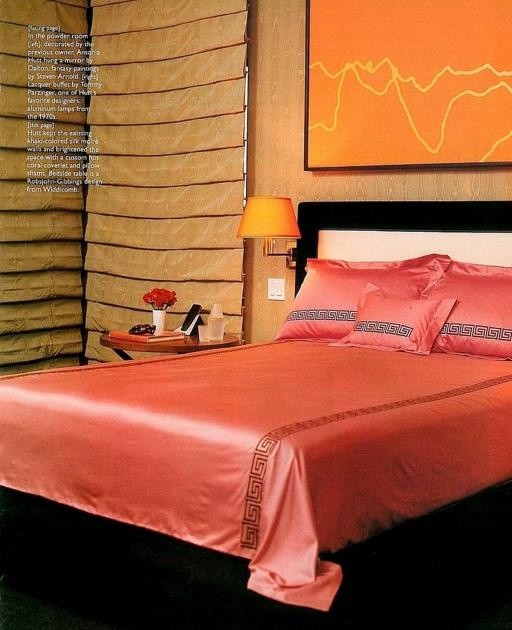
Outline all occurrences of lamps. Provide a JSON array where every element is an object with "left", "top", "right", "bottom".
[{"left": 237, "top": 194, "right": 302, "bottom": 268}]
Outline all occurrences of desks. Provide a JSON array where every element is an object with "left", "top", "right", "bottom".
[{"left": 100, "top": 330, "right": 239, "bottom": 361}]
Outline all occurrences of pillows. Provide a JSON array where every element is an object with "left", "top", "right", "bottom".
[{"left": 274, "top": 254, "right": 512, "bottom": 362}]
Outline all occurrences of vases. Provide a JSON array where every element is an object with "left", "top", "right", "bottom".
[{"left": 152, "top": 309, "right": 167, "bottom": 335}]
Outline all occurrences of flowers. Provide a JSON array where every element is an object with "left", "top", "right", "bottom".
[{"left": 143, "top": 288, "right": 178, "bottom": 310}]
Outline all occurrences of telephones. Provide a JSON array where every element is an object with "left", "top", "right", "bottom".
[{"left": 174, "top": 304, "right": 204, "bottom": 336}]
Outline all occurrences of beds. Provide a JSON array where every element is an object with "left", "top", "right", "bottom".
[{"left": 2, "top": 200, "right": 512, "bottom": 628}]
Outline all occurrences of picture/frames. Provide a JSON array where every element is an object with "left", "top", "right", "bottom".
[{"left": 303, "top": 0, "right": 512, "bottom": 172}]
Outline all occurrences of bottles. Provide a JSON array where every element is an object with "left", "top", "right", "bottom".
[{"left": 208, "top": 304, "right": 225, "bottom": 341}]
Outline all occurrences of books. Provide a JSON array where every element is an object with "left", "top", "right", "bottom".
[{"left": 106, "top": 328, "right": 187, "bottom": 343}]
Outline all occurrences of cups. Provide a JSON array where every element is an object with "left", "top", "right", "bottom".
[{"left": 199, "top": 325, "right": 211, "bottom": 342}]
